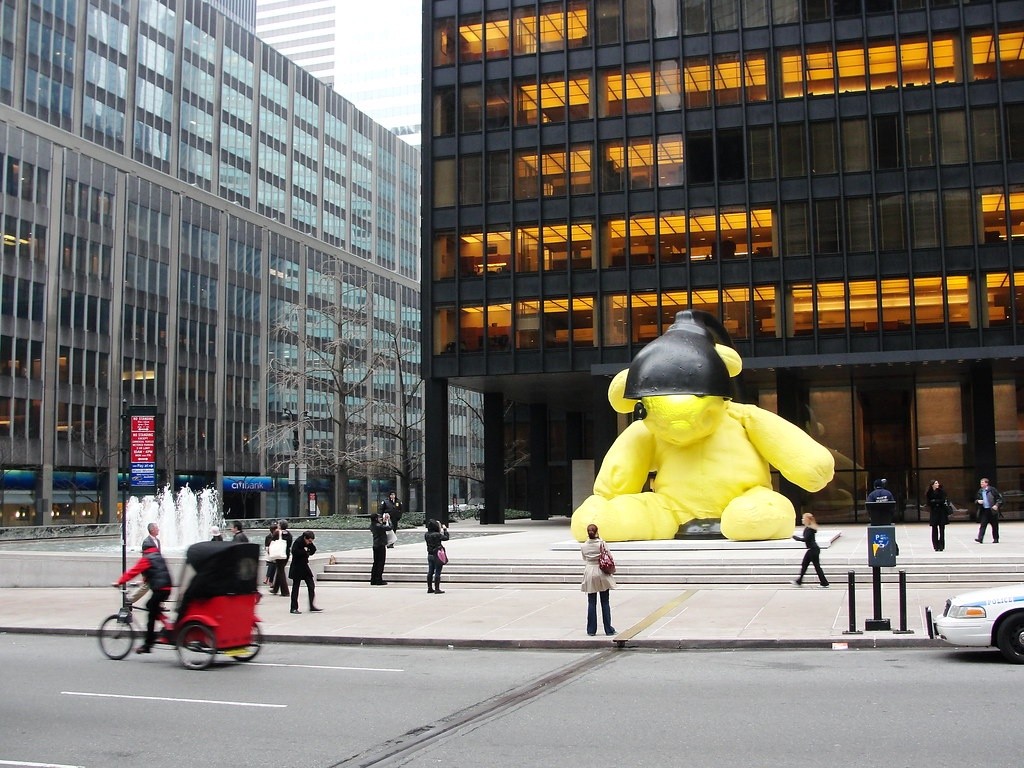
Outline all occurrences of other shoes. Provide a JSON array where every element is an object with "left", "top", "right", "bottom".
[
  {"left": 265, "top": 546, "right": 277, "bottom": 563},
  {"left": 589, "top": 633, "right": 594, "bottom": 636},
  {"left": 310, "top": 608, "right": 322, "bottom": 613},
  {"left": 791, "top": 579, "right": 802, "bottom": 588},
  {"left": 607, "top": 631, "right": 617, "bottom": 635},
  {"left": 975, "top": 538, "right": 982, "bottom": 543},
  {"left": 290, "top": 609, "right": 301, "bottom": 614},
  {"left": 992, "top": 539, "right": 998, "bottom": 543},
  {"left": 137, "top": 646, "right": 150, "bottom": 652},
  {"left": 817, "top": 585, "right": 828, "bottom": 589}
]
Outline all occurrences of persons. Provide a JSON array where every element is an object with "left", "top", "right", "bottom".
[
  {"left": 263, "top": 519, "right": 292, "bottom": 597},
  {"left": 865, "top": 480, "right": 899, "bottom": 556},
  {"left": 381, "top": 490, "right": 402, "bottom": 548},
  {"left": 792, "top": 513, "right": 829, "bottom": 588},
  {"left": 925, "top": 479, "right": 948, "bottom": 551},
  {"left": 124, "top": 523, "right": 170, "bottom": 612},
  {"left": 231, "top": 520, "right": 262, "bottom": 605},
  {"left": 975, "top": 478, "right": 1003, "bottom": 543},
  {"left": 209, "top": 525, "right": 223, "bottom": 541},
  {"left": 369, "top": 513, "right": 394, "bottom": 585},
  {"left": 112, "top": 541, "right": 172, "bottom": 654},
  {"left": 580, "top": 524, "right": 620, "bottom": 635},
  {"left": 288, "top": 531, "right": 324, "bottom": 615},
  {"left": 425, "top": 519, "right": 450, "bottom": 594}
]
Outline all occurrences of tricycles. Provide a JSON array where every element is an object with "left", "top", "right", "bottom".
[{"left": 98, "top": 541, "right": 266, "bottom": 672}]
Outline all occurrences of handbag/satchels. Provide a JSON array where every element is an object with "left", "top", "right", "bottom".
[
  {"left": 269, "top": 530, "right": 287, "bottom": 560},
  {"left": 434, "top": 547, "right": 448, "bottom": 564},
  {"left": 383, "top": 514, "right": 397, "bottom": 546},
  {"left": 599, "top": 542, "right": 616, "bottom": 575}
]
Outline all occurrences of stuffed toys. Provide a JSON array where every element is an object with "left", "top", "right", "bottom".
[{"left": 570, "top": 310, "right": 835, "bottom": 542}]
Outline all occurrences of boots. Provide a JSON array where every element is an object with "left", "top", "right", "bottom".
[
  {"left": 435, "top": 577, "right": 445, "bottom": 594},
  {"left": 427, "top": 576, "right": 435, "bottom": 593}
]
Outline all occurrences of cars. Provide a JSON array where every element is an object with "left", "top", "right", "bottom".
[{"left": 925, "top": 580, "right": 1024, "bottom": 665}]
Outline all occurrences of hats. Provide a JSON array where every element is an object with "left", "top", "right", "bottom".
[
  {"left": 142, "top": 543, "right": 156, "bottom": 550},
  {"left": 874, "top": 479, "right": 886, "bottom": 489},
  {"left": 210, "top": 526, "right": 220, "bottom": 537}
]
